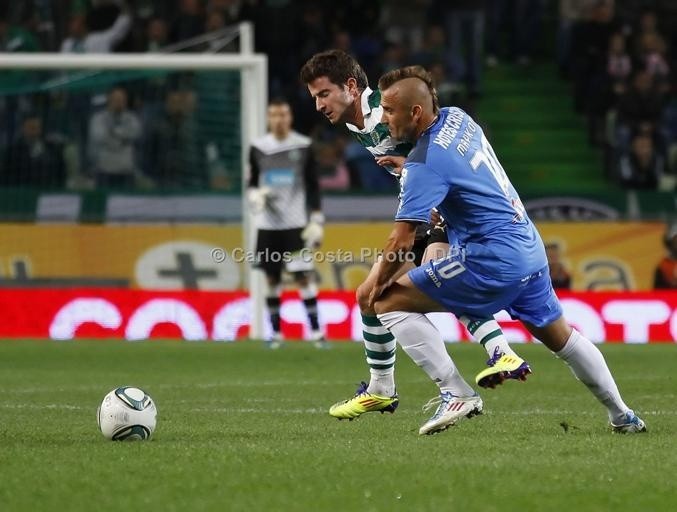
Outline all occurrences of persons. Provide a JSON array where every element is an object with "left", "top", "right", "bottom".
[
  {"left": 243, "top": 97, "right": 333, "bottom": 354},
  {"left": 0, "top": 1, "right": 676, "bottom": 222},
  {"left": 298, "top": 48, "right": 540, "bottom": 430},
  {"left": 652, "top": 221, "right": 677, "bottom": 290},
  {"left": 352, "top": 62, "right": 652, "bottom": 437},
  {"left": 543, "top": 242, "right": 574, "bottom": 291}
]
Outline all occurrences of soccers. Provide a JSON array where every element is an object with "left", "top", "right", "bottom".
[{"left": 96, "top": 386, "right": 158, "bottom": 442}]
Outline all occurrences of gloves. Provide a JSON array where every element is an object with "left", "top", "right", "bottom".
[
  {"left": 248, "top": 185, "right": 273, "bottom": 214},
  {"left": 301, "top": 213, "right": 326, "bottom": 253}
]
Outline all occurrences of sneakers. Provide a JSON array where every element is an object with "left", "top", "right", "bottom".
[
  {"left": 475, "top": 353, "right": 533, "bottom": 388},
  {"left": 418, "top": 390, "right": 483, "bottom": 436},
  {"left": 609, "top": 410, "right": 649, "bottom": 433},
  {"left": 328, "top": 382, "right": 399, "bottom": 422}
]
